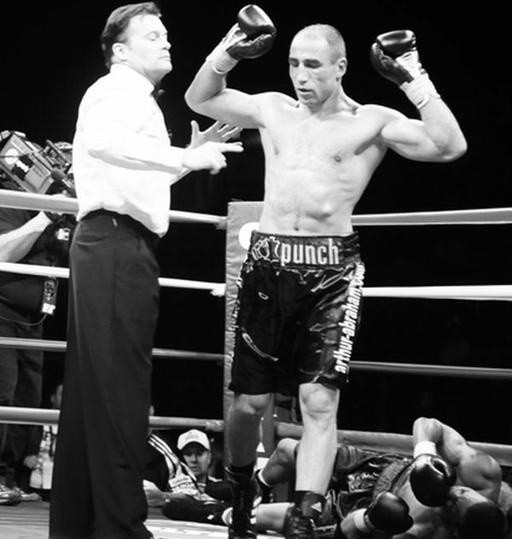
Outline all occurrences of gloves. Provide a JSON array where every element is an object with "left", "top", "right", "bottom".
[
  {"left": 370, "top": 28, "right": 442, "bottom": 110},
  {"left": 410, "top": 440, "right": 457, "bottom": 507},
  {"left": 205, "top": 4, "right": 278, "bottom": 76},
  {"left": 353, "top": 490, "right": 414, "bottom": 536}
]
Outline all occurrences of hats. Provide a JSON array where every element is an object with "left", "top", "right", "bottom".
[{"left": 176, "top": 429, "right": 211, "bottom": 452}]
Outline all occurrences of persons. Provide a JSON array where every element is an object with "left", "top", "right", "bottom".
[
  {"left": 29, "top": 383, "right": 65, "bottom": 501},
  {"left": 184, "top": 3, "right": 469, "bottom": 538},
  {"left": 49, "top": 2, "right": 245, "bottom": 539},
  {"left": 0, "top": 141, "right": 76, "bottom": 506},
  {"left": 142, "top": 406, "right": 511, "bottom": 538}
]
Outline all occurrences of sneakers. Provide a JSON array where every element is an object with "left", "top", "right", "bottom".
[{"left": 0, "top": 480, "right": 43, "bottom": 506}]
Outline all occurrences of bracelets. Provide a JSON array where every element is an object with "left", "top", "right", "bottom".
[{"left": 43, "top": 210, "right": 63, "bottom": 223}]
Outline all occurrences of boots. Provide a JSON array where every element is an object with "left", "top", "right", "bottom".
[
  {"left": 162, "top": 467, "right": 273, "bottom": 538},
  {"left": 283, "top": 491, "right": 328, "bottom": 539}
]
